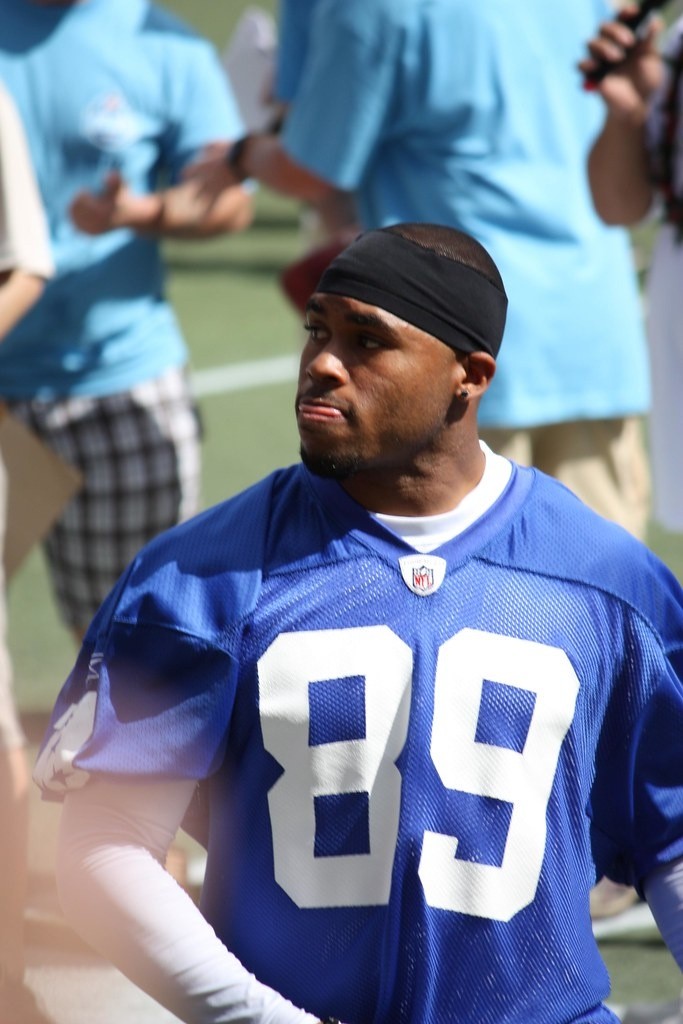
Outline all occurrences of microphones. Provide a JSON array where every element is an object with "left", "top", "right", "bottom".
[{"left": 582, "top": 0, "right": 674, "bottom": 91}]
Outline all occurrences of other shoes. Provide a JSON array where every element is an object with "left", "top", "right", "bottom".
[
  {"left": 591, "top": 901, "right": 665, "bottom": 945},
  {"left": 588, "top": 876, "right": 637, "bottom": 918}
]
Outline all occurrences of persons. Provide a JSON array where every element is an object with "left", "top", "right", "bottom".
[
  {"left": 0, "top": 0, "right": 683, "bottom": 1024},
  {"left": 33, "top": 224, "right": 683, "bottom": 1024}
]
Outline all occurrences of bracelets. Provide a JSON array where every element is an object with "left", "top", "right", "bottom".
[
  {"left": 269, "top": 120, "right": 282, "bottom": 134},
  {"left": 228, "top": 136, "right": 251, "bottom": 181}
]
